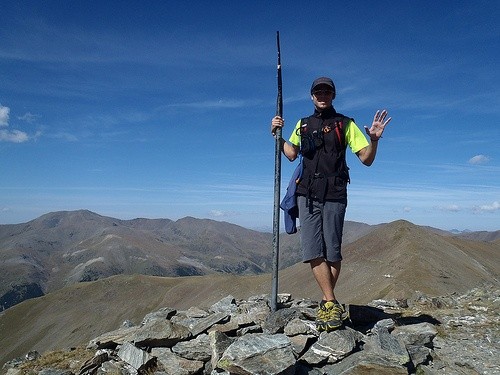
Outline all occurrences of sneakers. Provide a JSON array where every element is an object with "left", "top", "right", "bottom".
[
  {"left": 324, "top": 301, "right": 349, "bottom": 333},
  {"left": 315, "top": 303, "right": 328, "bottom": 331}
]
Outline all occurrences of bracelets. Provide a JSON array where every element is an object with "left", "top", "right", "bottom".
[{"left": 370, "top": 138, "right": 379, "bottom": 141}]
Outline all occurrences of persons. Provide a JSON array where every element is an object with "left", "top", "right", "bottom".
[{"left": 270, "top": 76, "right": 392, "bottom": 331}]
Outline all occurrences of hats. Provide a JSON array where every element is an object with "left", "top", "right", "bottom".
[{"left": 312, "top": 76, "right": 335, "bottom": 90}]
